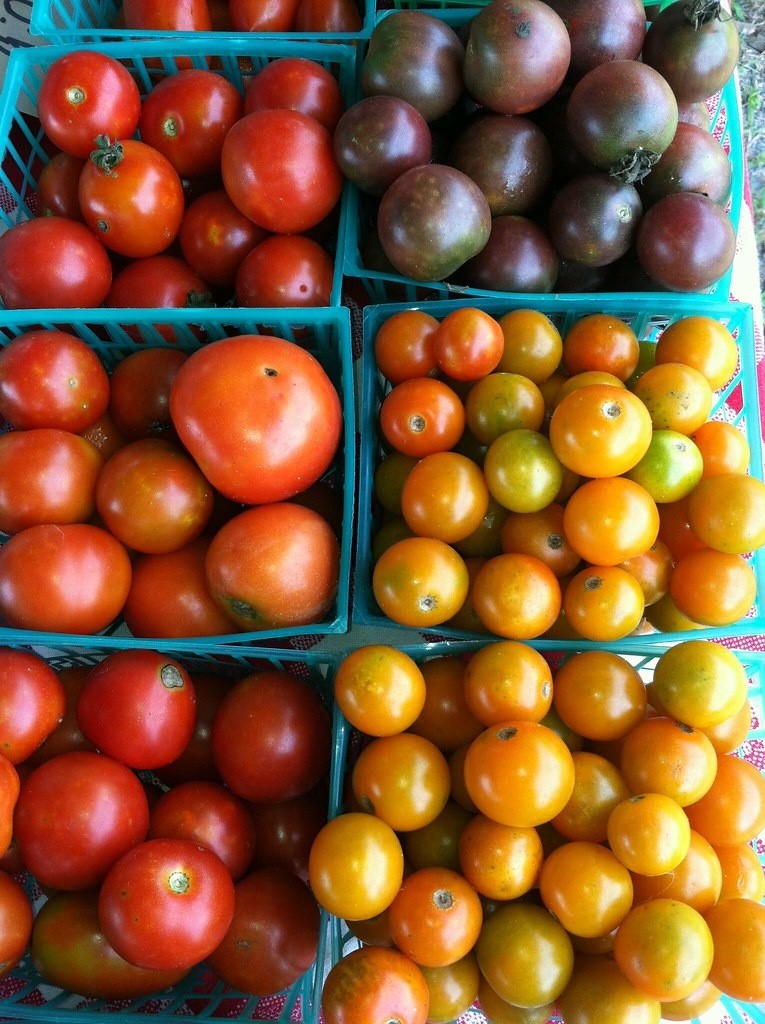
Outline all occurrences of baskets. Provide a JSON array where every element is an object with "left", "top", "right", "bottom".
[{"left": 2, "top": 0, "right": 762, "bottom": 1023}]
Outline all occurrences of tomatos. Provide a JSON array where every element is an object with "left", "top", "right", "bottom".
[{"left": 0, "top": 0, "right": 765, "bottom": 1024}]
